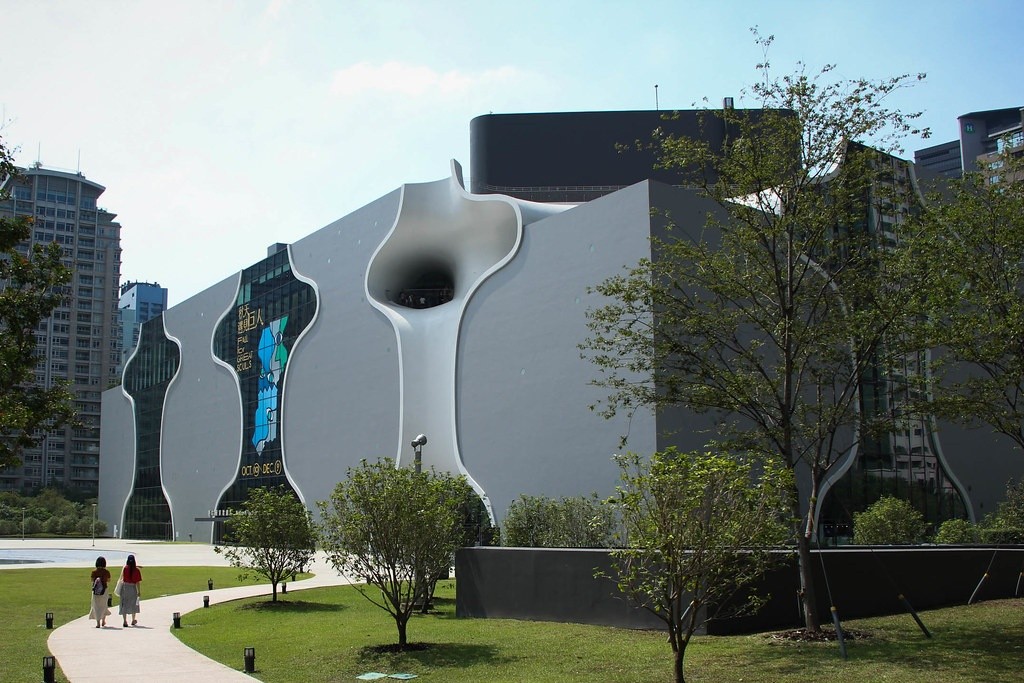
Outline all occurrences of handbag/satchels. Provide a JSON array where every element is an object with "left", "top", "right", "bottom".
[{"left": 114, "top": 579, "right": 124, "bottom": 596}]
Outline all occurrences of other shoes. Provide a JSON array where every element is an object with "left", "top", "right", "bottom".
[
  {"left": 102, "top": 622, "right": 106, "bottom": 626},
  {"left": 123, "top": 624, "right": 128, "bottom": 627},
  {"left": 96, "top": 626, "right": 100, "bottom": 628},
  {"left": 131, "top": 620, "right": 137, "bottom": 625}
]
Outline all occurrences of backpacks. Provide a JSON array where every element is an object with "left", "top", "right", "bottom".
[{"left": 94, "top": 577, "right": 105, "bottom": 595}]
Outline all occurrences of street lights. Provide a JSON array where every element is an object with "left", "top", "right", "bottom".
[
  {"left": 21, "top": 508, "right": 25, "bottom": 542},
  {"left": 92, "top": 504, "right": 95, "bottom": 546},
  {"left": 394, "top": 433, "right": 437, "bottom": 610}
]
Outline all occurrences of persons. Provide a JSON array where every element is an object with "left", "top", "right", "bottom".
[
  {"left": 89, "top": 557, "right": 111, "bottom": 628},
  {"left": 118, "top": 555, "right": 143, "bottom": 627}
]
